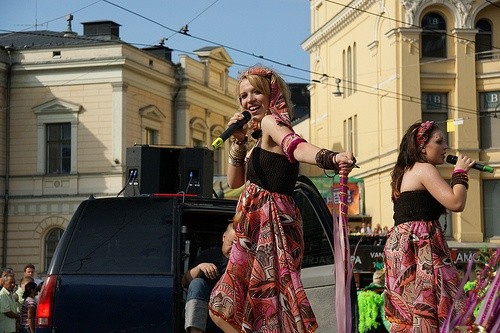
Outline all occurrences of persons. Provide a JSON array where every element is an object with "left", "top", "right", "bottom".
[
  {"left": 0, "top": 264, "right": 44, "bottom": 333},
  {"left": 180, "top": 222, "right": 236, "bottom": 333},
  {"left": 346, "top": 222, "right": 389, "bottom": 237},
  {"left": 379, "top": 118, "right": 478, "bottom": 333},
  {"left": 205, "top": 64, "right": 356, "bottom": 333}
]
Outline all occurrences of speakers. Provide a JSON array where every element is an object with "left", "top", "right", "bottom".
[{"left": 125, "top": 142, "right": 214, "bottom": 199}]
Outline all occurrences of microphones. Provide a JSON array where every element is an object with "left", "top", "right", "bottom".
[
  {"left": 212, "top": 111, "right": 251, "bottom": 150},
  {"left": 446, "top": 154, "right": 494, "bottom": 173}
]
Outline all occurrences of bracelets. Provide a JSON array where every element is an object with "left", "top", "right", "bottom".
[
  {"left": 228, "top": 134, "right": 248, "bottom": 169},
  {"left": 451, "top": 169, "right": 469, "bottom": 190},
  {"left": 314, "top": 149, "right": 342, "bottom": 178}
]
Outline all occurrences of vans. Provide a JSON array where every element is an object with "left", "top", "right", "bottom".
[{"left": 32, "top": 172, "right": 336, "bottom": 333}]
